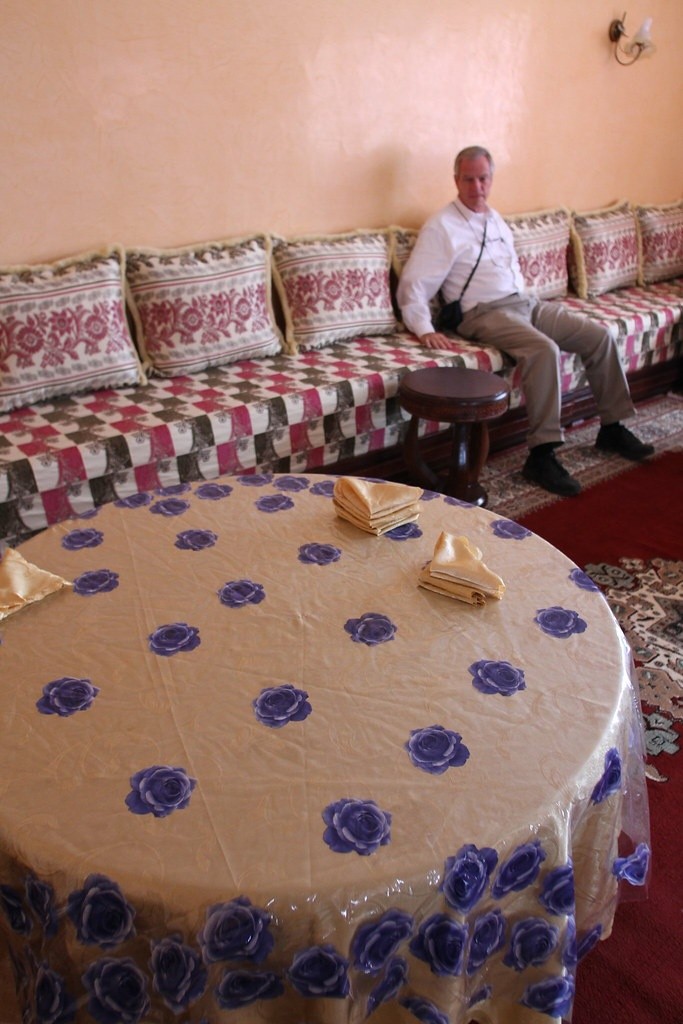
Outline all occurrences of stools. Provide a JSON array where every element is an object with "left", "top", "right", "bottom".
[{"left": 398, "top": 366, "right": 513, "bottom": 508}]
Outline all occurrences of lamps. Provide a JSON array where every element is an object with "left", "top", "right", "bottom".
[{"left": 609, "top": 11, "right": 658, "bottom": 68}]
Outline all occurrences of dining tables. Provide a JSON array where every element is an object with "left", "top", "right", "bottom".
[{"left": 0, "top": 471, "right": 652, "bottom": 1024}]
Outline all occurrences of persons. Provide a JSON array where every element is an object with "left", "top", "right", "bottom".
[{"left": 396, "top": 146, "right": 655, "bottom": 496}]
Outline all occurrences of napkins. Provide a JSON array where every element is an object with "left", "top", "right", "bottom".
[
  {"left": 0, "top": 545, "right": 75, "bottom": 621},
  {"left": 417, "top": 529, "right": 506, "bottom": 608},
  {"left": 331, "top": 476, "right": 425, "bottom": 536}
]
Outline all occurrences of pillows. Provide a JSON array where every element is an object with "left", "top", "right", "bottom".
[
  {"left": 0, "top": 242, "right": 147, "bottom": 415},
  {"left": 630, "top": 197, "right": 683, "bottom": 288},
  {"left": 390, "top": 224, "right": 452, "bottom": 329},
  {"left": 499, "top": 204, "right": 574, "bottom": 302},
  {"left": 124, "top": 231, "right": 293, "bottom": 379},
  {"left": 565, "top": 196, "right": 645, "bottom": 300},
  {"left": 267, "top": 225, "right": 407, "bottom": 356}
]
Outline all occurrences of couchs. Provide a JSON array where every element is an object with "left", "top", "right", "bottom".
[
  {"left": 499, "top": 238, "right": 683, "bottom": 413},
  {"left": 0, "top": 260, "right": 508, "bottom": 546}
]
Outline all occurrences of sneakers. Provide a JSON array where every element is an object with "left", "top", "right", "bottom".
[
  {"left": 594, "top": 425, "right": 654, "bottom": 454},
  {"left": 521, "top": 451, "right": 580, "bottom": 496}
]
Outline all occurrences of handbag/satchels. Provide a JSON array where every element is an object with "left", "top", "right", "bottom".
[{"left": 439, "top": 301, "right": 463, "bottom": 329}]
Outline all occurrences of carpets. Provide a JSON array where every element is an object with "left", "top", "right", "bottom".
[{"left": 398, "top": 388, "right": 683, "bottom": 1023}]
